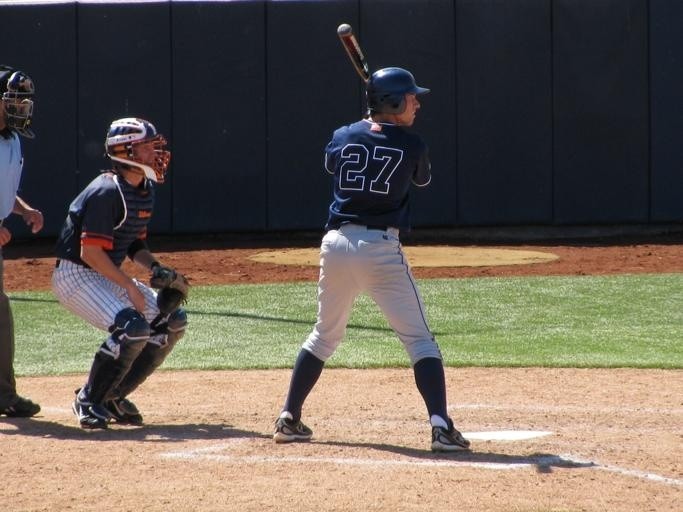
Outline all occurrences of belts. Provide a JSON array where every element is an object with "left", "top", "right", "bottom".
[
  {"left": 326, "top": 223, "right": 388, "bottom": 233},
  {"left": 50, "top": 258, "right": 92, "bottom": 271}
]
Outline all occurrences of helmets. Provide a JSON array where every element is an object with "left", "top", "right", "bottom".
[
  {"left": 367, "top": 68, "right": 429, "bottom": 115},
  {"left": 104, "top": 116, "right": 171, "bottom": 184},
  {"left": 0, "top": 66, "right": 34, "bottom": 140}
]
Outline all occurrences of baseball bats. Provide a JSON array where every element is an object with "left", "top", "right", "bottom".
[{"left": 337, "top": 24, "right": 372, "bottom": 83}]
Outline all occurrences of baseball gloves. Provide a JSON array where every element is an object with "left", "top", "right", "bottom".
[{"left": 150, "top": 268, "right": 189, "bottom": 315}]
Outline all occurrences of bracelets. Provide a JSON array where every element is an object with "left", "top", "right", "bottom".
[{"left": 150, "top": 261, "right": 160, "bottom": 270}]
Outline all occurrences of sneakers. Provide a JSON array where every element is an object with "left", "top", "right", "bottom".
[
  {"left": 272, "top": 417, "right": 312, "bottom": 442},
  {"left": 70, "top": 395, "right": 107, "bottom": 431},
  {"left": 428, "top": 422, "right": 470, "bottom": 453},
  {"left": 105, "top": 393, "right": 143, "bottom": 424},
  {"left": 0, "top": 394, "right": 40, "bottom": 418}
]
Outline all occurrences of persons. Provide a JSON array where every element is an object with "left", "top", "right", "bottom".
[
  {"left": 50, "top": 117, "right": 188, "bottom": 428},
  {"left": 0, "top": 65, "right": 43, "bottom": 418},
  {"left": 273, "top": 67, "right": 471, "bottom": 451}
]
